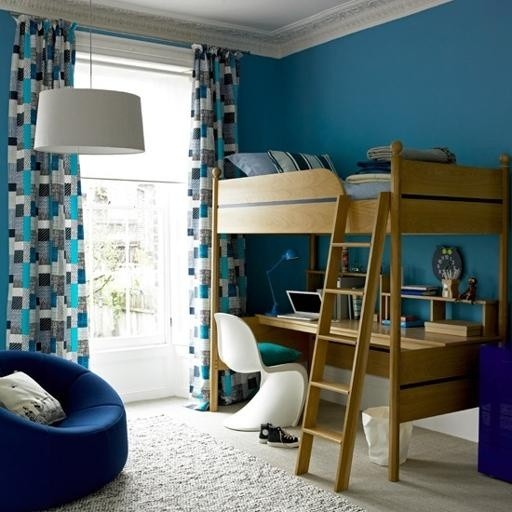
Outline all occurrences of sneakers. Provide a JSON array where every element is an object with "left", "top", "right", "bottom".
[
  {"left": 267, "top": 425, "right": 299, "bottom": 448},
  {"left": 259, "top": 424, "right": 272, "bottom": 444}
]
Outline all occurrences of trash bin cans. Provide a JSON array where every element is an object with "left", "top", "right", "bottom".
[{"left": 362, "top": 406, "right": 413, "bottom": 465}]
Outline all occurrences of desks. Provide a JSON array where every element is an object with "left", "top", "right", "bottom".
[{"left": 253, "top": 311, "right": 483, "bottom": 381}]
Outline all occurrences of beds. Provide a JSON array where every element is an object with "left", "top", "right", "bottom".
[{"left": 212, "top": 141, "right": 511, "bottom": 482}]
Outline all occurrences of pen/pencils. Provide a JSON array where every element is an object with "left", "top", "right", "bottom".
[{"left": 442, "top": 269, "right": 456, "bottom": 279}]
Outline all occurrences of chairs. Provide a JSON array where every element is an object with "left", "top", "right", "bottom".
[
  {"left": 0, "top": 346, "right": 130, "bottom": 512},
  {"left": 213, "top": 311, "right": 310, "bottom": 431}
]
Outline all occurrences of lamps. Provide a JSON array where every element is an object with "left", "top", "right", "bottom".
[
  {"left": 32, "top": 0, "right": 148, "bottom": 158},
  {"left": 263, "top": 248, "right": 301, "bottom": 316}
]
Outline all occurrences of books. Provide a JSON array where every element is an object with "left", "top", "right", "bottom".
[{"left": 332, "top": 277, "right": 442, "bottom": 327}]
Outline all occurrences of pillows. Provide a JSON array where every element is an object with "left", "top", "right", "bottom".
[
  {"left": 257, "top": 341, "right": 305, "bottom": 367},
  {"left": 223, "top": 148, "right": 340, "bottom": 177},
  {"left": 0, "top": 369, "right": 68, "bottom": 426}
]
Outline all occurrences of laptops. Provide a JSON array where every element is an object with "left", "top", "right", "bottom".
[{"left": 277, "top": 290, "right": 322, "bottom": 321}]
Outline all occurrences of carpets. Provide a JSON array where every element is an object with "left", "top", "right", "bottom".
[{"left": 40, "top": 411, "right": 367, "bottom": 512}]
